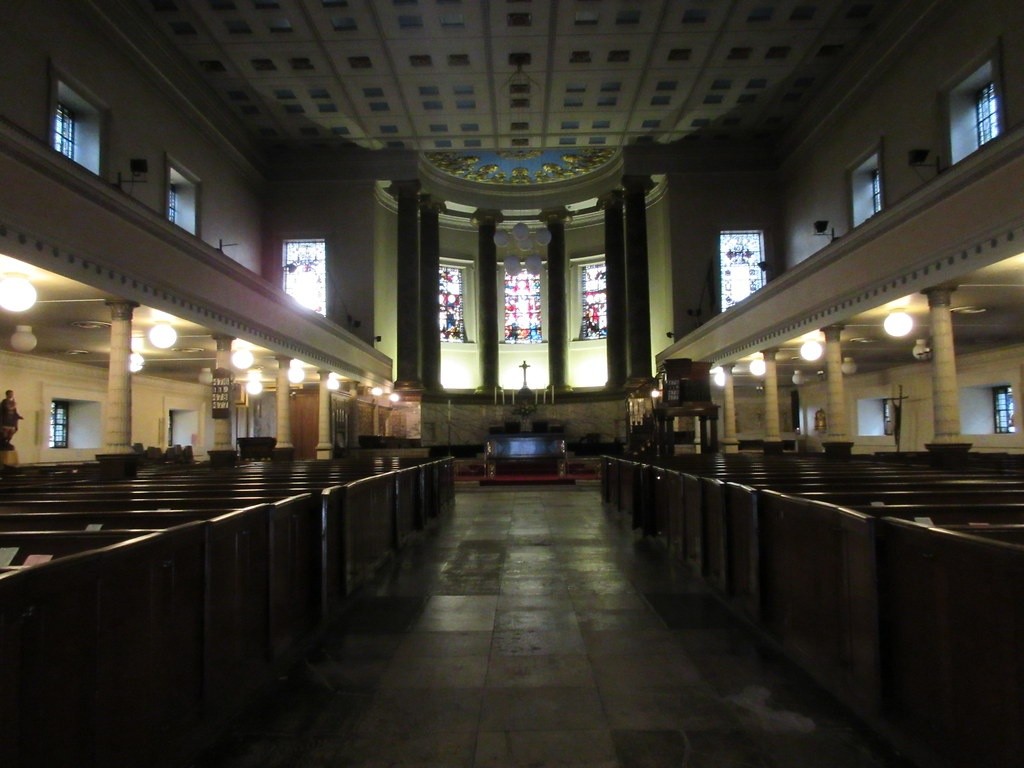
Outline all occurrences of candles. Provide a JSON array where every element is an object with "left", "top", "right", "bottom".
[
  {"left": 447, "top": 400, "right": 451, "bottom": 422},
  {"left": 552, "top": 385, "right": 554, "bottom": 404},
  {"left": 543, "top": 391, "right": 546, "bottom": 405},
  {"left": 501, "top": 387, "right": 505, "bottom": 405},
  {"left": 494, "top": 387, "right": 497, "bottom": 405},
  {"left": 535, "top": 389, "right": 537, "bottom": 405},
  {"left": 512, "top": 389, "right": 514, "bottom": 405}
]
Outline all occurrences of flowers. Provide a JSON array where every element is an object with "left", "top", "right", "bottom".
[{"left": 510, "top": 399, "right": 537, "bottom": 418}]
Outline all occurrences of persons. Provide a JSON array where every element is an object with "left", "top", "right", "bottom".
[{"left": 0, "top": 390, "right": 23, "bottom": 450}]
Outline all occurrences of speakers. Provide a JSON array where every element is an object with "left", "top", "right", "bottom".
[
  {"left": 907, "top": 149, "right": 929, "bottom": 163},
  {"left": 815, "top": 220, "right": 829, "bottom": 233},
  {"left": 666, "top": 332, "right": 672, "bottom": 338},
  {"left": 757, "top": 261, "right": 769, "bottom": 271},
  {"left": 288, "top": 263, "right": 297, "bottom": 273},
  {"left": 130, "top": 159, "right": 147, "bottom": 173},
  {"left": 376, "top": 336, "right": 381, "bottom": 341},
  {"left": 353, "top": 320, "right": 359, "bottom": 327}
]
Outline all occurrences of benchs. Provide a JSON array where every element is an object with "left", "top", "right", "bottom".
[
  {"left": 0, "top": 456, "right": 454, "bottom": 768},
  {"left": 599, "top": 451, "right": 1024, "bottom": 768}
]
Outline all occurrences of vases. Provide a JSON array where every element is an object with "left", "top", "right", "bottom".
[{"left": 520, "top": 415, "right": 534, "bottom": 432}]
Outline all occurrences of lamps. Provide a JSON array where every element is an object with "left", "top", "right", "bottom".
[
  {"left": 117, "top": 159, "right": 148, "bottom": 189},
  {"left": 715, "top": 310, "right": 931, "bottom": 385},
  {"left": 129, "top": 307, "right": 400, "bottom": 402},
  {"left": 199, "top": 367, "right": 213, "bottom": 385},
  {"left": 757, "top": 262, "right": 784, "bottom": 274},
  {"left": 666, "top": 332, "right": 675, "bottom": 338},
  {"left": 0, "top": 271, "right": 37, "bottom": 312},
  {"left": 11, "top": 325, "right": 37, "bottom": 352},
  {"left": 493, "top": 63, "right": 552, "bottom": 275},
  {"left": 908, "top": 149, "right": 941, "bottom": 174},
  {"left": 814, "top": 220, "right": 834, "bottom": 239}
]
